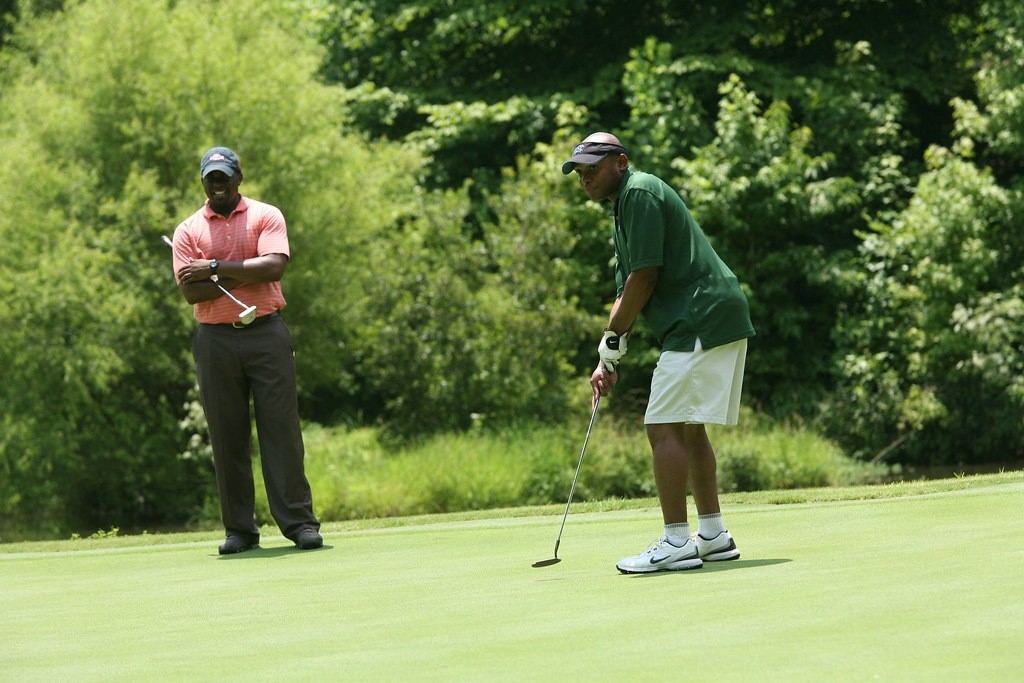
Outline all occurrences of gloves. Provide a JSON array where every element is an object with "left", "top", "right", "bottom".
[{"left": 597, "top": 328, "right": 628, "bottom": 371}]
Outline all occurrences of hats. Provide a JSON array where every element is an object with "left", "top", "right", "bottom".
[
  {"left": 562, "top": 142, "right": 631, "bottom": 175},
  {"left": 201, "top": 147, "right": 241, "bottom": 182}
]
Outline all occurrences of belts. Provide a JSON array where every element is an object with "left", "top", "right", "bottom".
[{"left": 200, "top": 310, "right": 280, "bottom": 329}]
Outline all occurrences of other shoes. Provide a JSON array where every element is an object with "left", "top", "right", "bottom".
[
  {"left": 219, "top": 535, "right": 259, "bottom": 554},
  {"left": 295, "top": 527, "right": 323, "bottom": 549}
]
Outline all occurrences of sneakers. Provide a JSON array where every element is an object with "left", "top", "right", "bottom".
[
  {"left": 692, "top": 529, "right": 740, "bottom": 561},
  {"left": 616, "top": 536, "right": 703, "bottom": 574}
]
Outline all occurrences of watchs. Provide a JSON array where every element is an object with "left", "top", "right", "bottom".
[{"left": 210, "top": 260, "right": 219, "bottom": 273}]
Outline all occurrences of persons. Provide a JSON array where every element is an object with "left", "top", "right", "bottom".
[
  {"left": 171, "top": 145, "right": 323, "bottom": 555},
  {"left": 562, "top": 131, "right": 757, "bottom": 574}
]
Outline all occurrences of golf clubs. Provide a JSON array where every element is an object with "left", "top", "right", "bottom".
[
  {"left": 531, "top": 399, "right": 599, "bottom": 568},
  {"left": 161, "top": 235, "right": 258, "bottom": 325}
]
